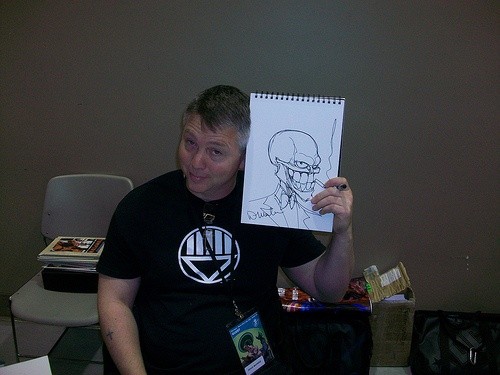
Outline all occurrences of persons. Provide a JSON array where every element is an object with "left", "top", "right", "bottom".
[{"left": 95, "top": 85, "right": 354, "bottom": 375}]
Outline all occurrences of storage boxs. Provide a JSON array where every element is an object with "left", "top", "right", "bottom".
[
  {"left": 41, "top": 264, "right": 99, "bottom": 293},
  {"left": 277, "top": 260, "right": 418, "bottom": 368}
]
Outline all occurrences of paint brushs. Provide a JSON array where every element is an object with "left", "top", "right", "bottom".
[{"left": 336, "top": 182, "right": 347, "bottom": 192}]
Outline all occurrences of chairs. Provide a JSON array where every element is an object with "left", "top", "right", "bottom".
[{"left": 9, "top": 174, "right": 135, "bottom": 375}]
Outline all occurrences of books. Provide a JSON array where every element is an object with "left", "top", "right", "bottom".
[{"left": 37, "top": 235, "right": 106, "bottom": 270}]
